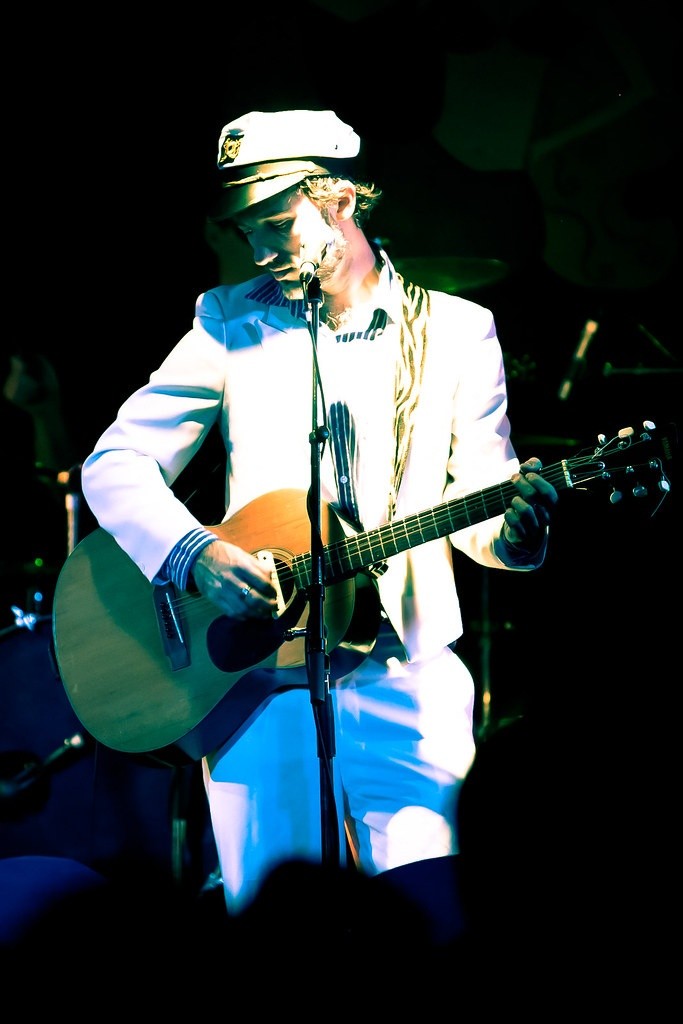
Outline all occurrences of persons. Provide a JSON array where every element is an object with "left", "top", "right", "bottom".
[{"left": 81, "top": 108, "right": 557, "bottom": 907}]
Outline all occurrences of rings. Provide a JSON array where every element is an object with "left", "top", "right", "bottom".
[{"left": 240, "top": 586, "right": 251, "bottom": 599}]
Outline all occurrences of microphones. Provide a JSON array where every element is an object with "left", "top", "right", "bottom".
[
  {"left": 0, "top": 761, "right": 52, "bottom": 799},
  {"left": 297, "top": 223, "right": 335, "bottom": 286},
  {"left": 560, "top": 319, "right": 599, "bottom": 400}
]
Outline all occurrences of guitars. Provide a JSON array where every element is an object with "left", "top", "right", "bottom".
[{"left": 52, "top": 421, "right": 672, "bottom": 772}]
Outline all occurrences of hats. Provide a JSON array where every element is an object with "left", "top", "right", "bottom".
[{"left": 216, "top": 109, "right": 361, "bottom": 220}]
